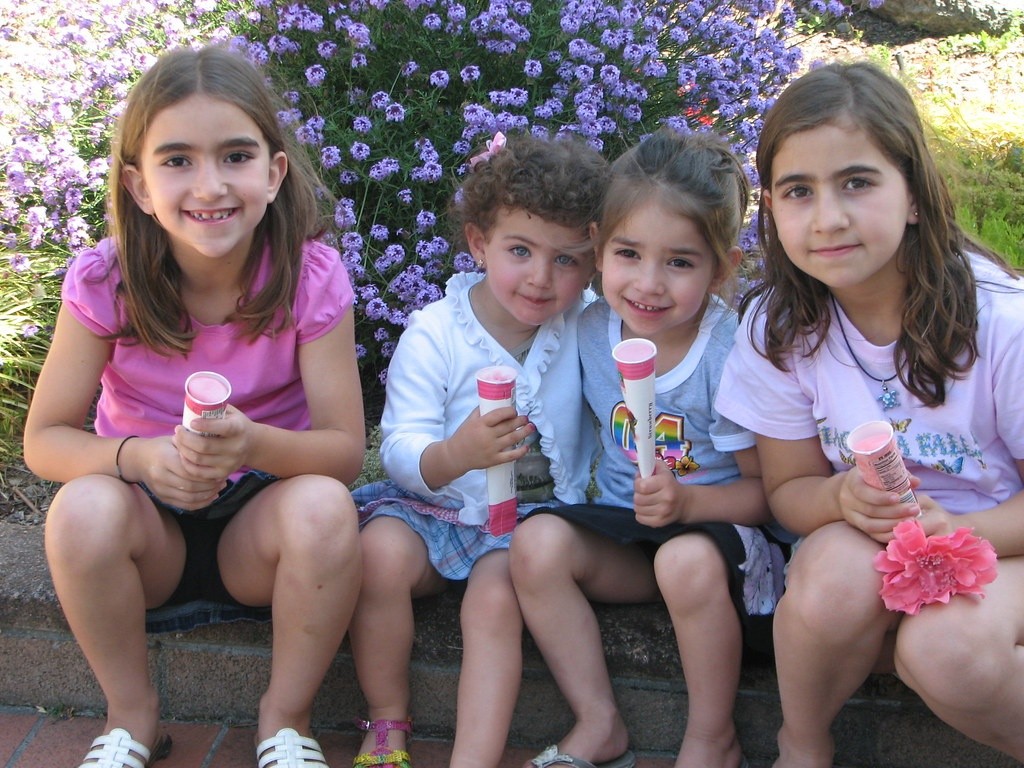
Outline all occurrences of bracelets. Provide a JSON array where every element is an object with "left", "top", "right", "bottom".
[{"left": 115, "top": 434, "right": 141, "bottom": 485}]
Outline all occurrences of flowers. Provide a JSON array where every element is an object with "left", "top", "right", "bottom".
[{"left": 874, "top": 514, "right": 1001, "bottom": 618}]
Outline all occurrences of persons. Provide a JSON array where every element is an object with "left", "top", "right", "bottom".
[
  {"left": 508, "top": 127, "right": 799, "bottom": 768},
  {"left": 332, "top": 132, "right": 612, "bottom": 767},
  {"left": 712, "top": 61, "right": 1023, "bottom": 768},
  {"left": 21, "top": 43, "right": 370, "bottom": 768}
]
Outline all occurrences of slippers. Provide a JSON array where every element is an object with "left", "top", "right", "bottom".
[
  {"left": 78, "top": 727, "right": 166, "bottom": 766},
  {"left": 532, "top": 740, "right": 638, "bottom": 766},
  {"left": 249, "top": 729, "right": 328, "bottom": 768}
]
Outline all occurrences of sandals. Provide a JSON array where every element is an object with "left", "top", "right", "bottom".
[{"left": 349, "top": 715, "right": 412, "bottom": 768}]
